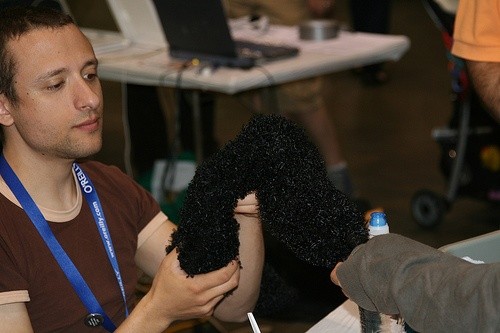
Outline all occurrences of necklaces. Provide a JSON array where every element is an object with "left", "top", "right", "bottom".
[{"left": 34, "top": 166, "right": 81, "bottom": 215}]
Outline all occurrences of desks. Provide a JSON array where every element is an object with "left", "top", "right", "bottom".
[{"left": 80, "top": 18, "right": 412, "bottom": 161}]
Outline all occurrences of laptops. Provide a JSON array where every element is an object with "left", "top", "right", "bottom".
[
  {"left": 154, "top": 0, "right": 300, "bottom": 70},
  {"left": 109, "top": 0, "right": 169, "bottom": 51}
]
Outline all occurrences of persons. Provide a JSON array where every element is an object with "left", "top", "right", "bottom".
[
  {"left": 329, "top": 0, "right": 500, "bottom": 332},
  {"left": 1, "top": 0, "right": 267, "bottom": 332},
  {"left": 331, "top": 0, "right": 397, "bottom": 86},
  {"left": 224, "top": 0, "right": 356, "bottom": 196}
]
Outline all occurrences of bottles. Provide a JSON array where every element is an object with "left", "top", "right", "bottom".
[{"left": 358, "top": 212, "right": 405, "bottom": 333}]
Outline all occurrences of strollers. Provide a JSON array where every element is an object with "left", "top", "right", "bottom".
[{"left": 409, "top": 0, "right": 500, "bottom": 230}]
